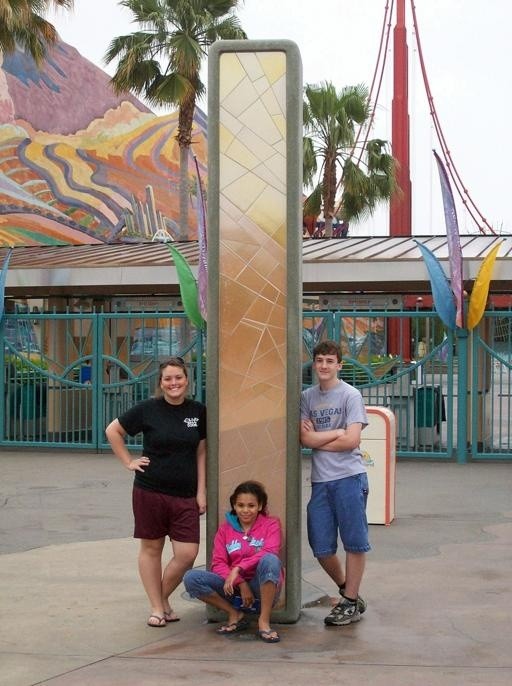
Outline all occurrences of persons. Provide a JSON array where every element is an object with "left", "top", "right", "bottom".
[
  {"left": 105, "top": 358, "right": 206, "bottom": 628},
  {"left": 184, "top": 481, "right": 284, "bottom": 643},
  {"left": 300, "top": 341, "right": 372, "bottom": 626}
]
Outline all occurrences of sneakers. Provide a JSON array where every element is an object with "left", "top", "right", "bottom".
[{"left": 323, "top": 588, "right": 368, "bottom": 626}]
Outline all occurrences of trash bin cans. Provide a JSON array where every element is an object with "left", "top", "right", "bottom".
[{"left": 414, "top": 384, "right": 443, "bottom": 447}]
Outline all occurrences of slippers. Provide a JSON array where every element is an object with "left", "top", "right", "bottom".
[
  {"left": 256, "top": 630, "right": 280, "bottom": 643},
  {"left": 147, "top": 609, "right": 180, "bottom": 627},
  {"left": 216, "top": 617, "right": 249, "bottom": 634}
]
